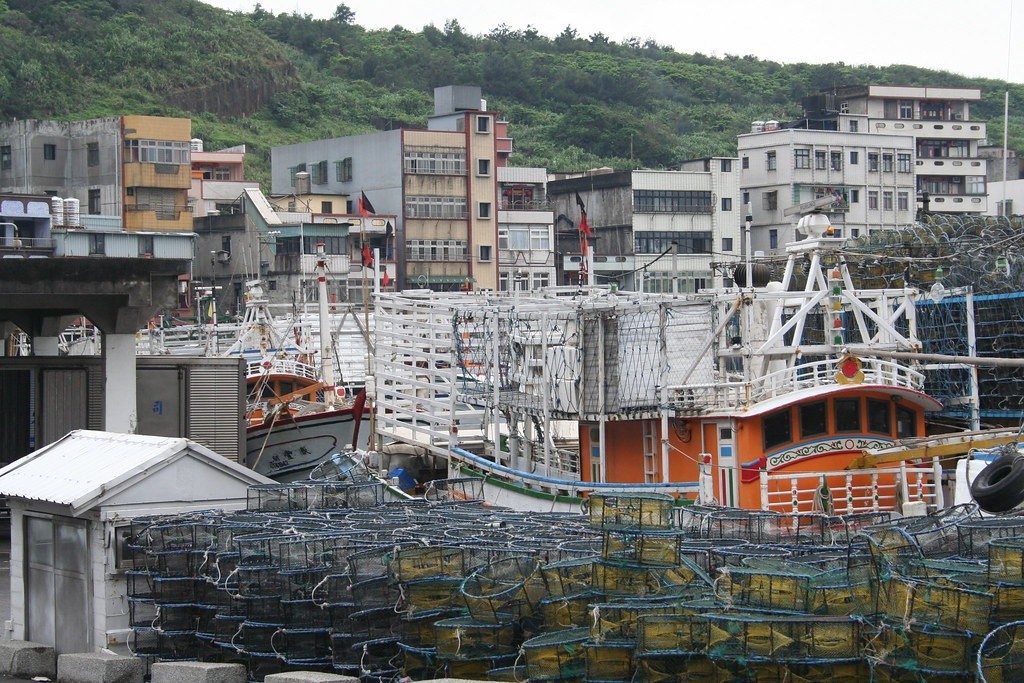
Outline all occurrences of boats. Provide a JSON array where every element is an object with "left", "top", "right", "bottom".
[
  {"left": 9, "top": 312, "right": 105, "bottom": 359},
  {"left": 136, "top": 204, "right": 373, "bottom": 493},
  {"left": 370, "top": 135, "right": 1024, "bottom": 553}
]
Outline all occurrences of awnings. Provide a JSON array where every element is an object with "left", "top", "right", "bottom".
[
  {"left": 800, "top": 183, "right": 852, "bottom": 189},
  {"left": 407, "top": 276, "right": 477, "bottom": 291}
]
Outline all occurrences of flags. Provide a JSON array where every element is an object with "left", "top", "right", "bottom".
[
  {"left": 383, "top": 272, "right": 389, "bottom": 287},
  {"left": 363, "top": 243, "right": 372, "bottom": 264},
  {"left": 359, "top": 199, "right": 368, "bottom": 219},
  {"left": 361, "top": 191, "right": 375, "bottom": 214},
  {"left": 575, "top": 191, "right": 592, "bottom": 253},
  {"left": 386, "top": 222, "right": 393, "bottom": 239}
]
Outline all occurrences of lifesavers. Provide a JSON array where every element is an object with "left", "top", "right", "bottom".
[{"left": 971, "top": 452, "right": 1024, "bottom": 512}]
